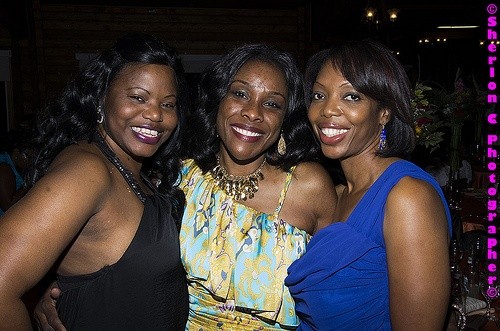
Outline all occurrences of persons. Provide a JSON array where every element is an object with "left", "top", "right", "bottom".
[
  {"left": 26, "top": 42, "right": 338, "bottom": 331},
  {"left": 0, "top": 46, "right": 189, "bottom": 331},
  {"left": 0, "top": 128, "right": 35, "bottom": 216},
  {"left": 285, "top": 42, "right": 453, "bottom": 331}
]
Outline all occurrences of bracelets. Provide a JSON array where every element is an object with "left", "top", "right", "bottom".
[{"left": 22, "top": 182, "right": 30, "bottom": 193}]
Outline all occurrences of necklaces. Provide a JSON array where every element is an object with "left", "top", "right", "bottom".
[{"left": 212, "top": 149, "right": 268, "bottom": 202}]
